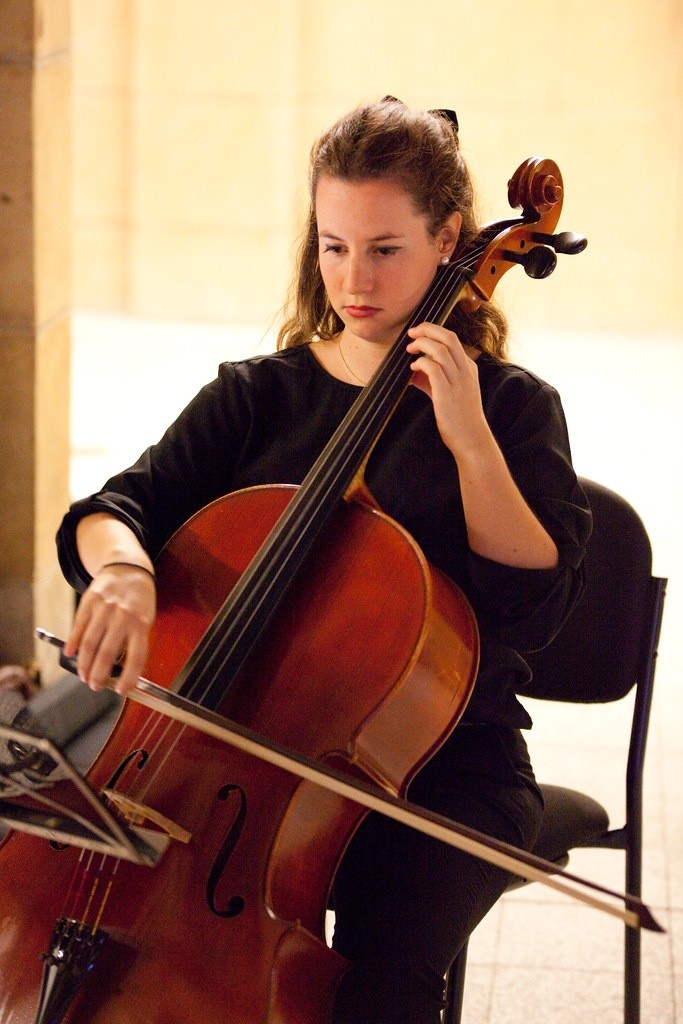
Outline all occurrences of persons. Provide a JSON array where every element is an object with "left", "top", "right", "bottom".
[{"left": 56, "top": 96, "right": 596, "bottom": 1024}]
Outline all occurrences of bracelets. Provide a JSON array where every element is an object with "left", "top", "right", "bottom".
[{"left": 98, "top": 560, "right": 158, "bottom": 580}]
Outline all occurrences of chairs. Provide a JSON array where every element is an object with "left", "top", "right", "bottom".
[{"left": 439, "top": 475, "right": 667, "bottom": 1024}]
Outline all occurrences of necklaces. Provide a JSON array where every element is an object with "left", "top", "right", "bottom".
[{"left": 338, "top": 334, "right": 369, "bottom": 387}]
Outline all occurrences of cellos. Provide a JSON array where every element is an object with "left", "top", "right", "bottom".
[{"left": 0, "top": 157, "right": 589, "bottom": 1023}]
